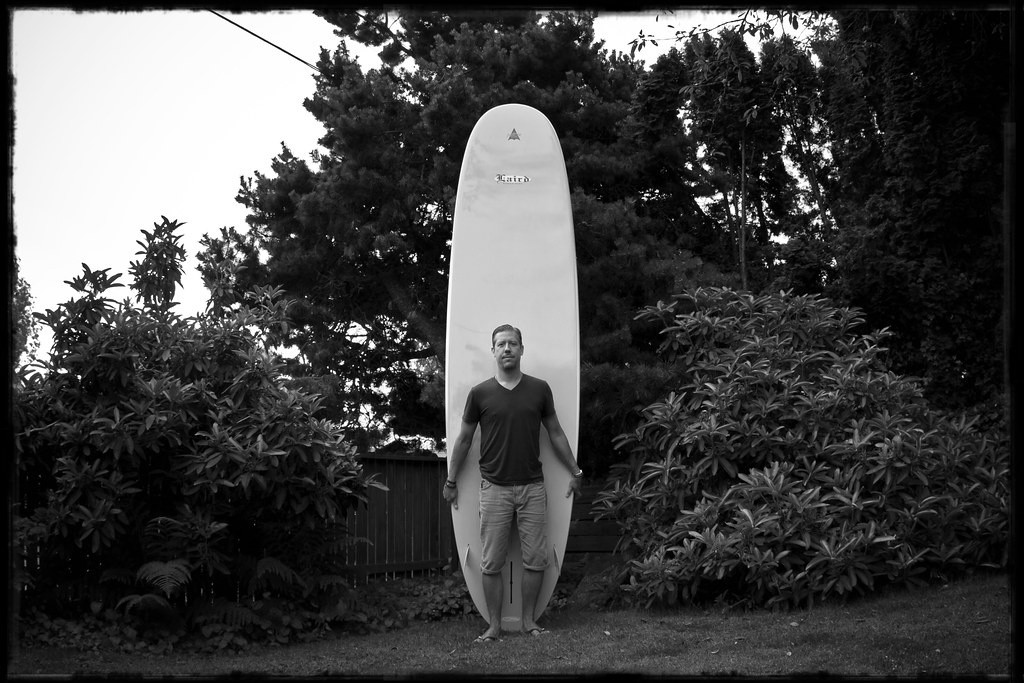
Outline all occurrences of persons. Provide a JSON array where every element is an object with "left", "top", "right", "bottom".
[{"left": 442, "top": 325, "right": 585, "bottom": 642}]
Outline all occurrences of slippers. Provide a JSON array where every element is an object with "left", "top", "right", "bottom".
[
  {"left": 473, "top": 633, "right": 502, "bottom": 643},
  {"left": 522, "top": 628, "right": 550, "bottom": 638}
]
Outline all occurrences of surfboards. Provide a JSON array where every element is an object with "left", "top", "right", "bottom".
[{"left": 442, "top": 102, "right": 585, "bottom": 633}]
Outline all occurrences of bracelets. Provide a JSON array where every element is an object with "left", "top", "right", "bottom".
[
  {"left": 574, "top": 470, "right": 583, "bottom": 478},
  {"left": 447, "top": 480, "right": 456, "bottom": 484},
  {"left": 446, "top": 483, "right": 456, "bottom": 488}
]
